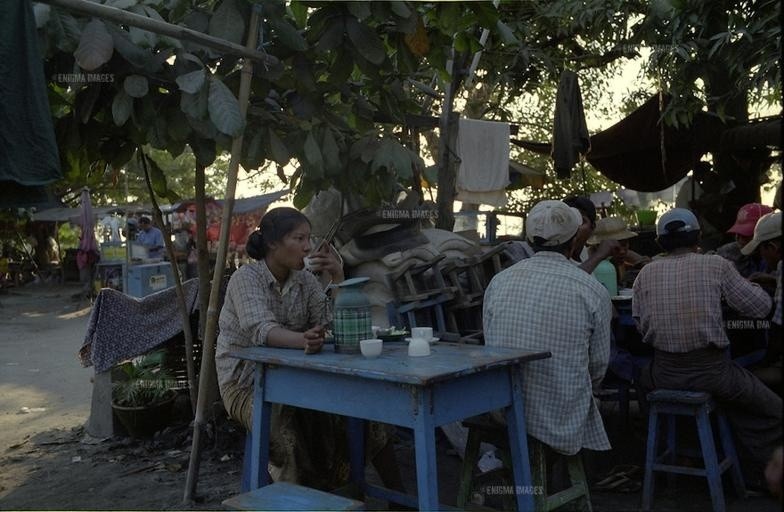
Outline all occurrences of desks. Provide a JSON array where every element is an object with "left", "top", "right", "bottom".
[
  {"left": 611, "top": 305, "right": 752, "bottom": 434},
  {"left": 231, "top": 339, "right": 552, "bottom": 512}
]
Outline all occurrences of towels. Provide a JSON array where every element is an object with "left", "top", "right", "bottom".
[{"left": 456, "top": 118, "right": 510, "bottom": 205}]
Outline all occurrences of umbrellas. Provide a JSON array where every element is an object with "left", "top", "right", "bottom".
[{"left": 77, "top": 187, "right": 97, "bottom": 302}]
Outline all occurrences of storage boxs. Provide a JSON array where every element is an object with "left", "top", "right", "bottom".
[{"left": 127, "top": 261, "right": 175, "bottom": 298}]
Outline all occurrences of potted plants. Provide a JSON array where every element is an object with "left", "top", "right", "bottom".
[{"left": 111, "top": 348, "right": 178, "bottom": 437}]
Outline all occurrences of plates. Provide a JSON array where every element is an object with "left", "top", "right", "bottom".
[
  {"left": 378, "top": 330, "right": 409, "bottom": 341},
  {"left": 405, "top": 337, "right": 440, "bottom": 345}
]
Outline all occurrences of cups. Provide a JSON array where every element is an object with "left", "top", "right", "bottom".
[
  {"left": 411, "top": 326, "right": 434, "bottom": 339},
  {"left": 360, "top": 339, "right": 383, "bottom": 359},
  {"left": 408, "top": 338, "right": 431, "bottom": 357},
  {"left": 303, "top": 256, "right": 321, "bottom": 270}
]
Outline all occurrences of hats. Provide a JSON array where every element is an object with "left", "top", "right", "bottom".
[
  {"left": 658, "top": 208, "right": 700, "bottom": 236},
  {"left": 728, "top": 202, "right": 782, "bottom": 255},
  {"left": 586, "top": 217, "right": 639, "bottom": 246},
  {"left": 525, "top": 200, "right": 583, "bottom": 248}
]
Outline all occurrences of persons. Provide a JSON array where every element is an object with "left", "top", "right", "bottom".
[
  {"left": 214, "top": 206, "right": 411, "bottom": 511},
  {"left": 121, "top": 210, "right": 196, "bottom": 259},
  {"left": 439, "top": 197, "right": 614, "bottom": 508},
  {"left": 560, "top": 161, "right": 783, "bottom": 501}
]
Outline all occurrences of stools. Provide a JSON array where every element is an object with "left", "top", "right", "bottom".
[
  {"left": 221, "top": 481, "right": 367, "bottom": 512},
  {"left": 457, "top": 413, "right": 592, "bottom": 512},
  {"left": 385, "top": 242, "right": 516, "bottom": 345},
  {"left": 640, "top": 389, "right": 747, "bottom": 512}
]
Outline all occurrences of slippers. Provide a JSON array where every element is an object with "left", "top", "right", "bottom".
[{"left": 594, "top": 463, "right": 641, "bottom": 494}]
